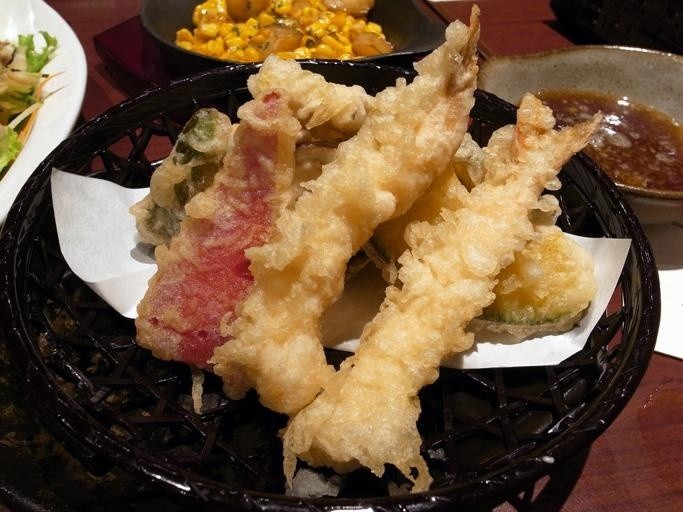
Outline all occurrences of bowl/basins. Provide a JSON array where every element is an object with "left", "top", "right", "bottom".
[{"left": 472, "top": 38, "right": 681, "bottom": 229}]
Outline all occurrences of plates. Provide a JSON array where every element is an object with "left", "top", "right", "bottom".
[
  {"left": 138, "top": 0, "right": 460, "bottom": 67},
  {"left": 0, "top": 0, "right": 86, "bottom": 226}
]
